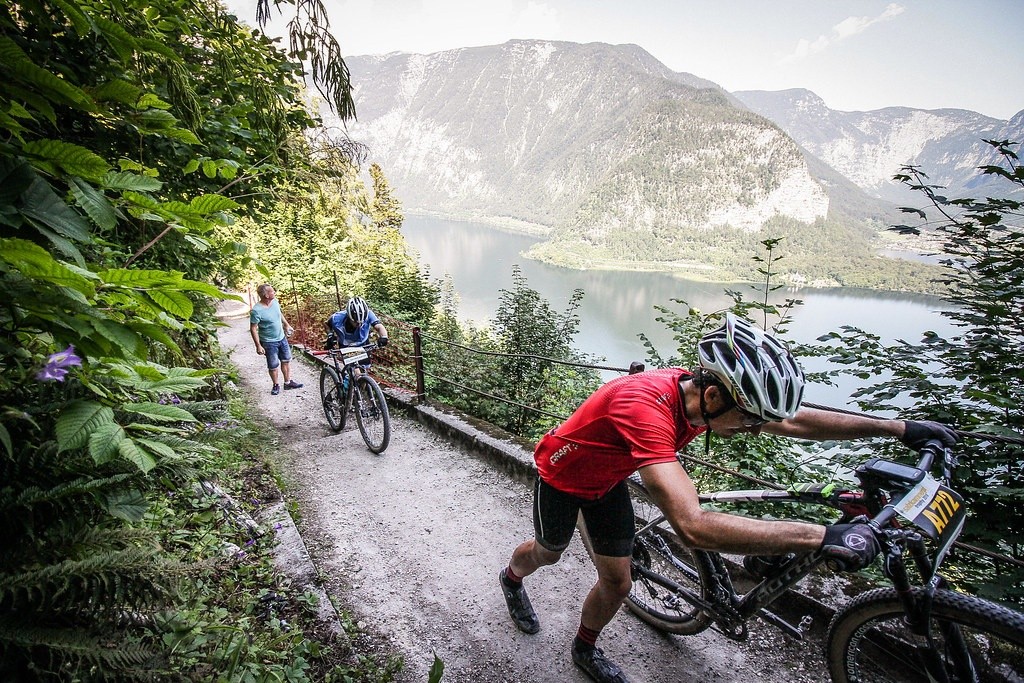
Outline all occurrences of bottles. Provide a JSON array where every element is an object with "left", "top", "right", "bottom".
[
  {"left": 744, "top": 550, "right": 792, "bottom": 577},
  {"left": 343, "top": 375, "right": 350, "bottom": 397}
]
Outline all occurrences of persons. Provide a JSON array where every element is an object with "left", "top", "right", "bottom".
[
  {"left": 498, "top": 310, "right": 960, "bottom": 683},
  {"left": 323, "top": 297, "right": 388, "bottom": 415},
  {"left": 249, "top": 284, "right": 303, "bottom": 395}
]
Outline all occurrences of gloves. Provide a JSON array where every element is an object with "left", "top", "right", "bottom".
[
  {"left": 378, "top": 337, "right": 388, "bottom": 346},
  {"left": 327, "top": 333, "right": 336, "bottom": 349},
  {"left": 898, "top": 418, "right": 959, "bottom": 449},
  {"left": 820, "top": 522, "right": 883, "bottom": 572}
]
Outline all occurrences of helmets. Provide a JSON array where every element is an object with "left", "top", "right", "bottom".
[
  {"left": 347, "top": 296, "right": 368, "bottom": 324},
  {"left": 698, "top": 312, "right": 805, "bottom": 422}
]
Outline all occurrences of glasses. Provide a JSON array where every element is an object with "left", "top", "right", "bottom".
[{"left": 735, "top": 406, "right": 762, "bottom": 428}]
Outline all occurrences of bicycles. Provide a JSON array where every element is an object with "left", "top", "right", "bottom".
[
  {"left": 310, "top": 343, "right": 393, "bottom": 453},
  {"left": 549, "top": 430, "right": 1024, "bottom": 683}
]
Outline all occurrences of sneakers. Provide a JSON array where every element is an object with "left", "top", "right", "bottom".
[
  {"left": 272, "top": 383, "right": 281, "bottom": 395},
  {"left": 283, "top": 381, "right": 303, "bottom": 390},
  {"left": 500, "top": 569, "right": 541, "bottom": 634},
  {"left": 570, "top": 638, "right": 627, "bottom": 683}
]
